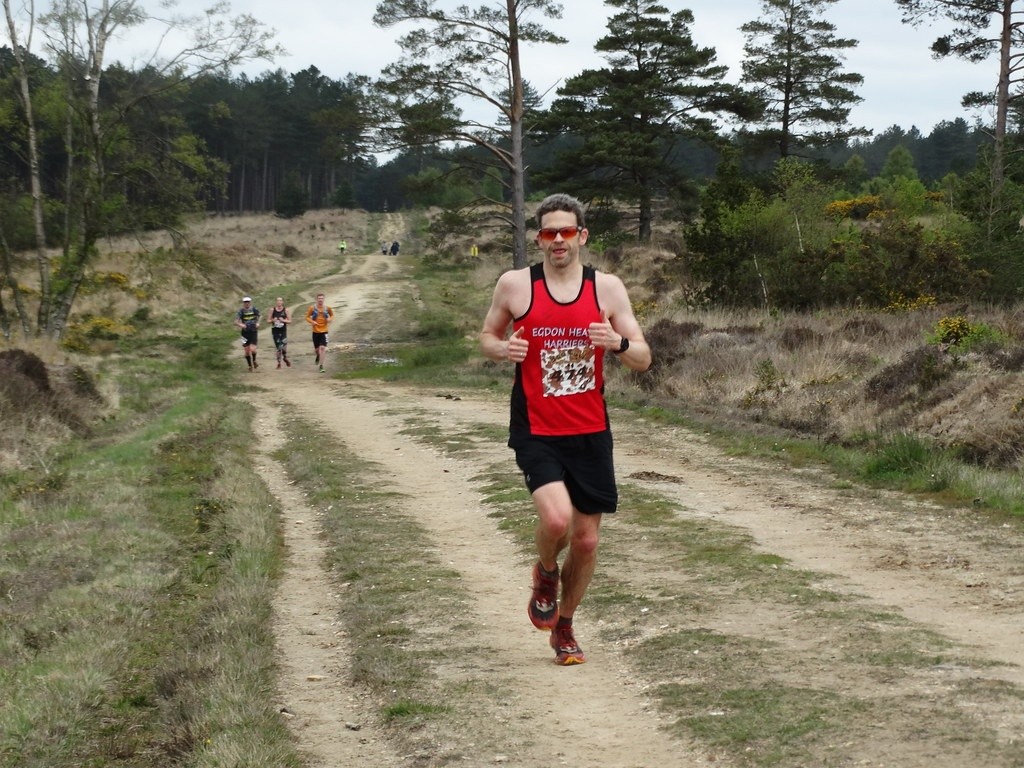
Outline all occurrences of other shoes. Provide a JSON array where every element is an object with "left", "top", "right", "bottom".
[
  {"left": 283, "top": 358, "right": 291, "bottom": 367},
  {"left": 253, "top": 361, "right": 259, "bottom": 368},
  {"left": 315, "top": 355, "right": 320, "bottom": 364},
  {"left": 319, "top": 364, "right": 325, "bottom": 372},
  {"left": 278, "top": 362, "right": 281, "bottom": 368},
  {"left": 248, "top": 363, "right": 253, "bottom": 372}
]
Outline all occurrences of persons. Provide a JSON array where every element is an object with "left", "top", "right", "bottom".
[
  {"left": 382, "top": 240, "right": 399, "bottom": 255},
  {"left": 305, "top": 293, "right": 334, "bottom": 374},
  {"left": 471, "top": 244, "right": 480, "bottom": 259},
  {"left": 267, "top": 296, "right": 292, "bottom": 371},
  {"left": 338, "top": 239, "right": 346, "bottom": 253},
  {"left": 481, "top": 192, "right": 652, "bottom": 666},
  {"left": 233, "top": 297, "right": 261, "bottom": 372}
]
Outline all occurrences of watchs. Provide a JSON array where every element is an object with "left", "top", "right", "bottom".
[{"left": 613, "top": 335, "right": 629, "bottom": 354}]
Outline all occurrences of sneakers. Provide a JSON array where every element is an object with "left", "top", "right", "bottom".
[
  {"left": 526, "top": 560, "right": 558, "bottom": 630},
  {"left": 549, "top": 622, "right": 586, "bottom": 664}
]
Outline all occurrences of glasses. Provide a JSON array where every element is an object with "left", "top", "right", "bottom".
[{"left": 538, "top": 226, "right": 582, "bottom": 241}]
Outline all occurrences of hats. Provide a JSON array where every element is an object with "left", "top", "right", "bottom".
[{"left": 243, "top": 297, "right": 251, "bottom": 301}]
